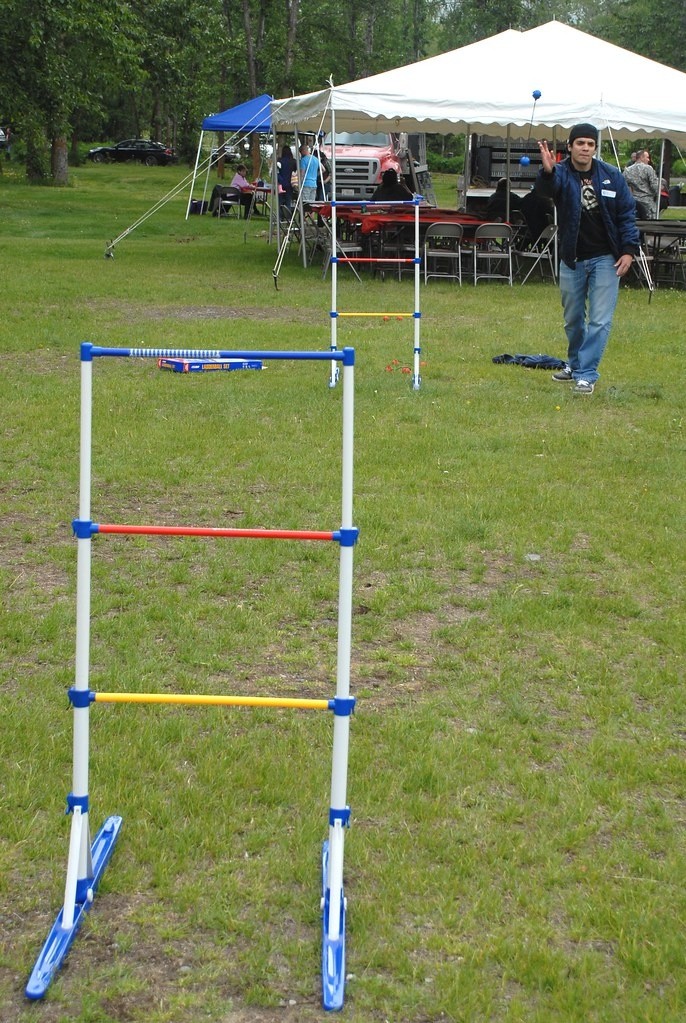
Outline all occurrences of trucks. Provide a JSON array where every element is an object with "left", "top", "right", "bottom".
[
  {"left": 457, "top": 132, "right": 571, "bottom": 221},
  {"left": 316, "top": 133, "right": 428, "bottom": 200},
  {"left": 245, "top": 136, "right": 301, "bottom": 160}
]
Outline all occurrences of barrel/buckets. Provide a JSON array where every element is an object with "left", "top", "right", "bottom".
[{"left": 668, "top": 186, "right": 686, "bottom": 206}]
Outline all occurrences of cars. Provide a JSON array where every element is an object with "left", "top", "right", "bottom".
[
  {"left": 0, "top": 127, "right": 8, "bottom": 150},
  {"left": 88, "top": 140, "right": 178, "bottom": 167},
  {"left": 212, "top": 145, "right": 241, "bottom": 163}
]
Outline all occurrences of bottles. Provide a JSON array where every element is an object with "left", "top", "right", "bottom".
[{"left": 361, "top": 199, "right": 367, "bottom": 213}]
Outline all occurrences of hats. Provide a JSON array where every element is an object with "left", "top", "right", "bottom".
[
  {"left": 569, "top": 123, "right": 598, "bottom": 150},
  {"left": 661, "top": 178, "right": 669, "bottom": 194}
]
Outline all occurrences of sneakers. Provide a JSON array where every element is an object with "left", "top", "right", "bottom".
[
  {"left": 552, "top": 364, "right": 575, "bottom": 382},
  {"left": 573, "top": 379, "right": 595, "bottom": 395}
]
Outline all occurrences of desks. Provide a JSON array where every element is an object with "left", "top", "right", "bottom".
[
  {"left": 241, "top": 182, "right": 276, "bottom": 222},
  {"left": 336, "top": 205, "right": 415, "bottom": 213},
  {"left": 635, "top": 220, "right": 686, "bottom": 228},
  {"left": 337, "top": 211, "right": 456, "bottom": 266},
  {"left": 634, "top": 225, "right": 686, "bottom": 289},
  {"left": 361, "top": 216, "right": 502, "bottom": 281},
  {"left": 348, "top": 213, "right": 478, "bottom": 273}
]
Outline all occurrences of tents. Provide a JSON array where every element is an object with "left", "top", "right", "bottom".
[
  {"left": 271, "top": 20, "right": 686, "bottom": 273},
  {"left": 185, "top": 94, "right": 325, "bottom": 221}
]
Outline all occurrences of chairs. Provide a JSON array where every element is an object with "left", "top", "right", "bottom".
[
  {"left": 218, "top": 186, "right": 241, "bottom": 220},
  {"left": 208, "top": 184, "right": 237, "bottom": 218},
  {"left": 280, "top": 203, "right": 686, "bottom": 289}
]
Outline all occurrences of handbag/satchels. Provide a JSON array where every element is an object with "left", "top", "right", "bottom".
[{"left": 492, "top": 353, "right": 568, "bottom": 369}]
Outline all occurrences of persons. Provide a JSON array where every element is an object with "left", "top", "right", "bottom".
[
  {"left": 224, "top": 164, "right": 264, "bottom": 220},
  {"left": 368, "top": 169, "right": 413, "bottom": 201},
  {"left": 275, "top": 146, "right": 298, "bottom": 220},
  {"left": 486, "top": 179, "right": 521, "bottom": 221},
  {"left": 534, "top": 124, "right": 642, "bottom": 397},
  {"left": 297, "top": 147, "right": 326, "bottom": 226},
  {"left": 622, "top": 147, "right": 670, "bottom": 222},
  {"left": 312, "top": 150, "right": 333, "bottom": 225},
  {"left": 521, "top": 187, "right": 556, "bottom": 254}
]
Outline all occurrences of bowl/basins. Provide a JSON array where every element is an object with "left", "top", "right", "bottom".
[{"left": 351, "top": 210, "right": 361, "bottom": 214}]
